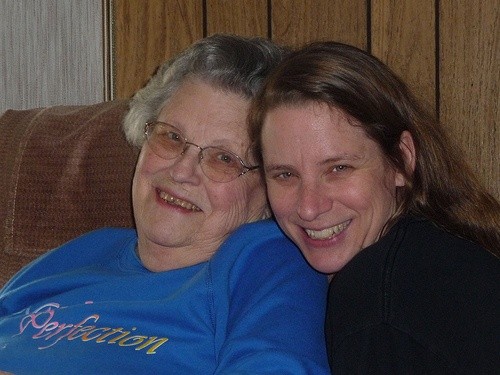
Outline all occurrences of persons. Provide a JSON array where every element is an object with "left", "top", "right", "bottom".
[
  {"left": 259, "top": 39, "right": 500, "bottom": 375},
  {"left": 0, "top": 29, "right": 330, "bottom": 375}
]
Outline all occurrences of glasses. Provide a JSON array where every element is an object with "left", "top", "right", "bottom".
[{"left": 140, "top": 119, "right": 262, "bottom": 183}]
